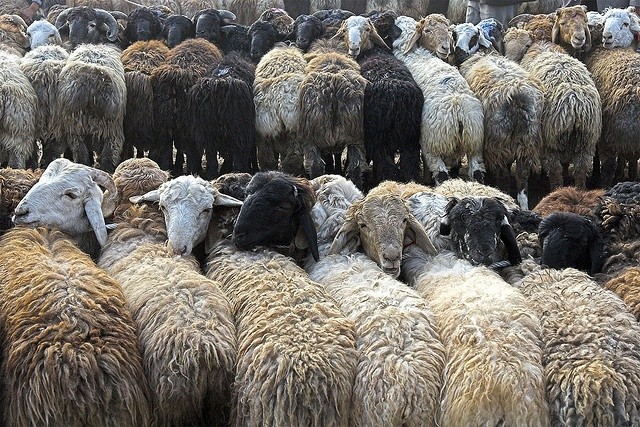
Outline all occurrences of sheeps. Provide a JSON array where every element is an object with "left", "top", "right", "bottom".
[{"left": 1, "top": 3, "right": 639, "bottom": 427}]
